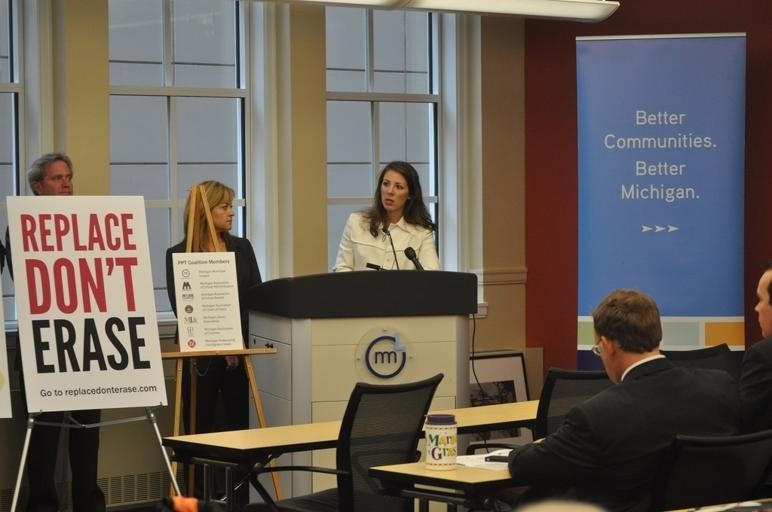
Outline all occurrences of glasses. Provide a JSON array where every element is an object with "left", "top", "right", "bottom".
[{"left": 592, "top": 344, "right": 601, "bottom": 356}]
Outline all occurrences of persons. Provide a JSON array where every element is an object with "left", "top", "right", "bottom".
[
  {"left": 510, "top": 289, "right": 739, "bottom": 506},
  {"left": 726, "top": 259, "right": 770, "bottom": 442},
  {"left": 168, "top": 179, "right": 263, "bottom": 509},
  {"left": 5, "top": 151, "right": 111, "bottom": 509},
  {"left": 330, "top": 160, "right": 442, "bottom": 274}
]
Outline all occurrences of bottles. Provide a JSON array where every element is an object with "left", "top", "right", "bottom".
[{"left": 423, "top": 413, "right": 458, "bottom": 472}]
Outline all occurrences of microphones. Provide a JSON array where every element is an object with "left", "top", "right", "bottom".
[
  {"left": 364, "top": 261, "right": 382, "bottom": 270},
  {"left": 382, "top": 228, "right": 400, "bottom": 270},
  {"left": 404, "top": 245, "right": 427, "bottom": 268}
]
